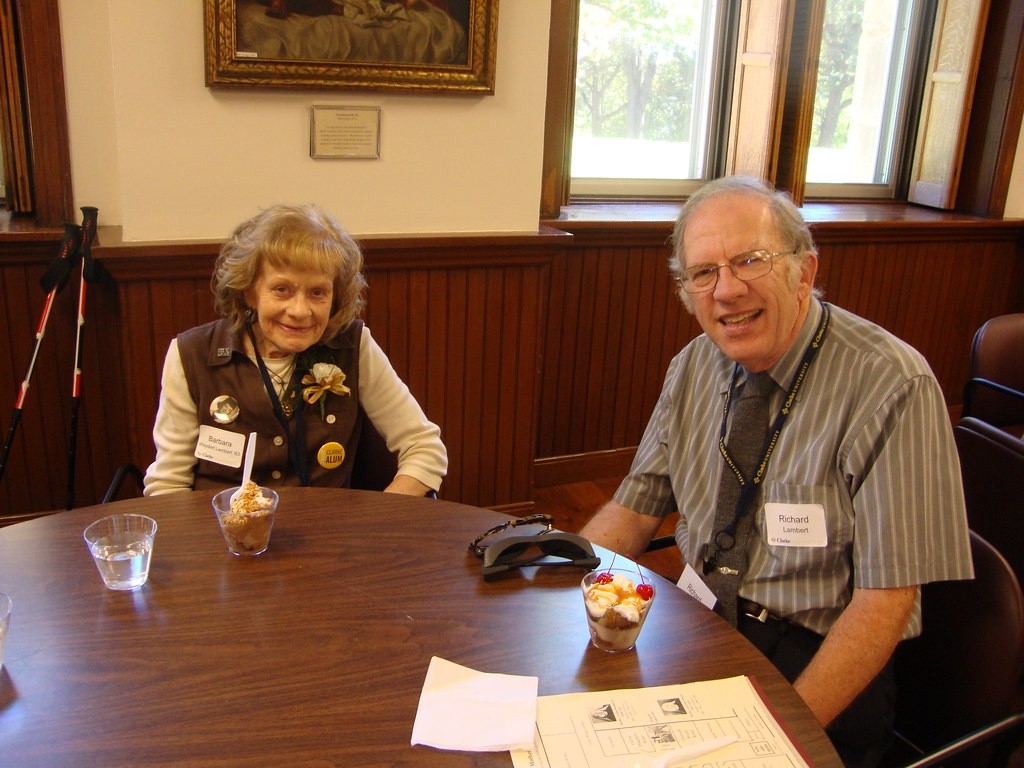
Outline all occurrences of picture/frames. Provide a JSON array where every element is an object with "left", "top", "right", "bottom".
[{"left": 203, "top": 0, "right": 499, "bottom": 95}]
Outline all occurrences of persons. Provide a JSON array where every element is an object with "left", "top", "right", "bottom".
[
  {"left": 211, "top": 397, "right": 237, "bottom": 423},
  {"left": 142, "top": 204, "right": 448, "bottom": 498},
  {"left": 577, "top": 175, "right": 973, "bottom": 768}
]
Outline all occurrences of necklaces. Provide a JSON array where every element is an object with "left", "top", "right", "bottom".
[{"left": 264, "top": 357, "right": 294, "bottom": 393}]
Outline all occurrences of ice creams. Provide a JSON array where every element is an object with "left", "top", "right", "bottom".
[
  {"left": 585, "top": 570, "right": 654, "bottom": 652},
  {"left": 221, "top": 478, "right": 274, "bottom": 555}
]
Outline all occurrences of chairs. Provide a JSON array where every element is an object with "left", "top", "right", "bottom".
[
  {"left": 954, "top": 313, "right": 1024, "bottom": 458},
  {"left": 893, "top": 527, "right": 1024, "bottom": 768}
]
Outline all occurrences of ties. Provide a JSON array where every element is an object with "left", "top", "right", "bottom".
[{"left": 703, "top": 371, "right": 779, "bottom": 629}]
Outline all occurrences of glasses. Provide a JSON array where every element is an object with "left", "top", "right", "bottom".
[
  {"left": 468, "top": 514, "right": 555, "bottom": 560},
  {"left": 674, "top": 249, "right": 803, "bottom": 293},
  {"left": 483, "top": 532, "right": 601, "bottom": 579}
]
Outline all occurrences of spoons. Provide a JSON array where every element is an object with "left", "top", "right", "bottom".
[{"left": 229, "top": 432, "right": 257, "bottom": 510}]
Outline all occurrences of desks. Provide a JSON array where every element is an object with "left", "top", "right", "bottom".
[{"left": 0, "top": 486, "right": 842, "bottom": 768}]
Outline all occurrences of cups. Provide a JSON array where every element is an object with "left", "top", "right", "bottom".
[
  {"left": 0, "top": 593, "right": 12, "bottom": 669},
  {"left": 212, "top": 486, "right": 279, "bottom": 555},
  {"left": 83, "top": 513, "right": 158, "bottom": 591},
  {"left": 581, "top": 569, "right": 656, "bottom": 652}
]
